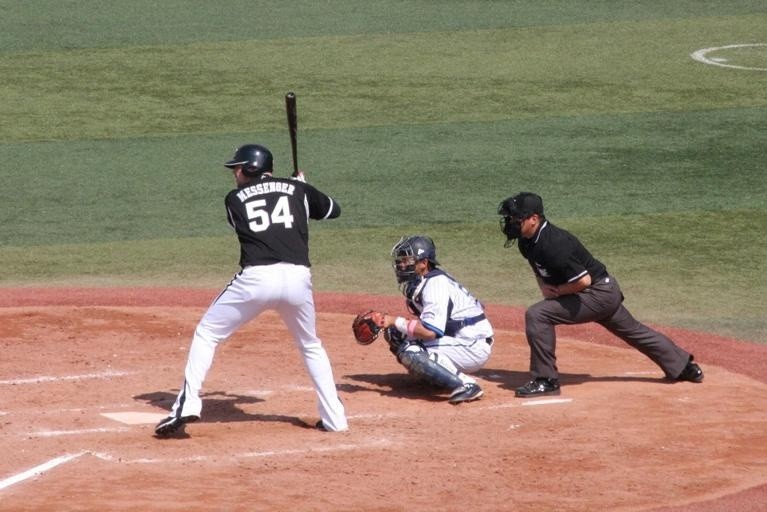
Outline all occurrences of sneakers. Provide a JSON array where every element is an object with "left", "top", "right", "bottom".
[
  {"left": 449, "top": 384, "right": 482, "bottom": 402},
  {"left": 315, "top": 419, "right": 326, "bottom": 431},
  {"left": 155, "top": 415, "right": 198, "bottom": 437},
  {"left": 516, "top": 374, "right": 561, "bottom": 398},
  {"left": 683, "top": 362, "right": 706, "bottom": 383}
]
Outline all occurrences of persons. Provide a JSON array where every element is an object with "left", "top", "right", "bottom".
[
  {"left": 496, "top": 193, "right": 705, "bottom": 398},
  {"left": 362, "top": 236, "right": 495, "bottom": 406},
  {"left": 155, "top": 144, "right": 350, "bottom": 439}
]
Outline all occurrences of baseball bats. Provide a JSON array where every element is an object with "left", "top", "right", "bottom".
[{"left": 285, "top": 92, "right": 299, "bottom": 175}]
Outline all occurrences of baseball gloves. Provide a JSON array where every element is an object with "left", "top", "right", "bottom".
[{"left": 352, "top": 310, "right": 382, "bottom": 345}]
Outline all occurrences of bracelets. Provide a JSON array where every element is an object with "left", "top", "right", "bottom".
[{"left": 394, "top": 315, "right": 417, "bottom": 337}]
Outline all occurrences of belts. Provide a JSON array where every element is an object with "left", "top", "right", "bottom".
[
  {"left": 486, "top": 336, "right": 493, "bottom": 345},
  {"left": 590, "top": 270, "right": 608, "bottom": 286}
]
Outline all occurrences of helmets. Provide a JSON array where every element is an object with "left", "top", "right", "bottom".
[
  {"left": 390, "top": 236, "right": 440, "bottom": 286},
  {"left": 224, "top": 145, "right": 272, "bottom": 176},
  {"left": 499, "top": 192, "right": 543, "bottom": 249}
]
